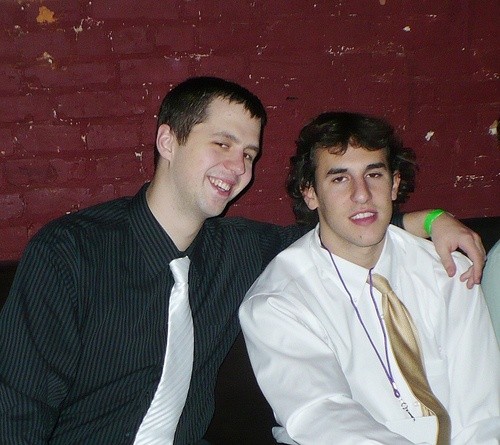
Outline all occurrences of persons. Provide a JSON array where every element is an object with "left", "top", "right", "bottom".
[
  {"left": 3, "top": 75, "right": 489, "bottom": 445},
  {"left": 237, "top": 110, "right": 498, "bottom": 445}
]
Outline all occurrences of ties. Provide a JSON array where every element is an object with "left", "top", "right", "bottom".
[
  {"left": 366, "top": 272, "right": 451, "bottom": 445},
  {"left": 132, "top": 256, "right": 195, "bottom": 445}
]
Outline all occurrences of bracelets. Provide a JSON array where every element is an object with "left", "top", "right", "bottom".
[{"left": 423, "top": 208, "right": 445, "bottom": 235}]
